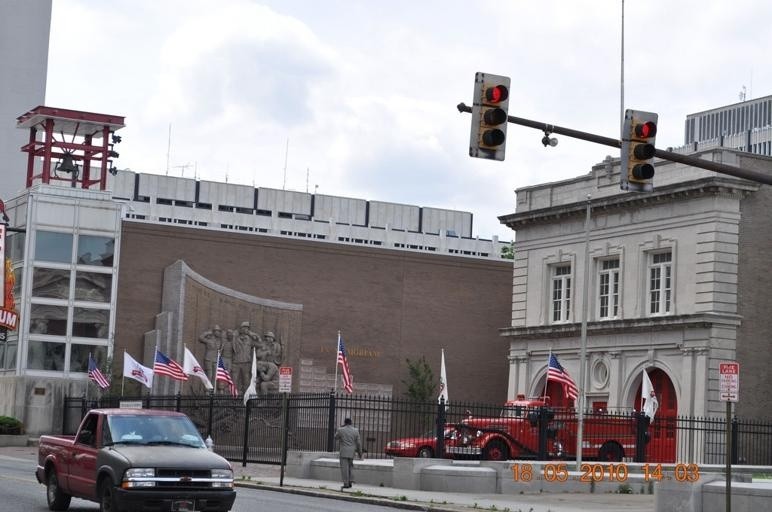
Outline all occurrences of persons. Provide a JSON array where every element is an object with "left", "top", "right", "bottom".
[
  {"left": 199, "top": 321, "right": 282, "bottom": 393},
  {"left": 335, "top": 418, "right": 362, "bottom": 488}
]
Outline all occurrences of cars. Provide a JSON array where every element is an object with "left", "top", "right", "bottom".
[{"left": 384, "top": 422, "right": 462, "bottom": 458}]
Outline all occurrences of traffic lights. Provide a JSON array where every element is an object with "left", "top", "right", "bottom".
[
  {"left": 478, "top": 84, "right": 508, "bottom": 149},
  {"left": 630, "top": 118, "right": 657, "bottom": 185}
]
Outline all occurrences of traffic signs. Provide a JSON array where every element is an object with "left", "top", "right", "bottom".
[{"left": 720, "top": 363, "right": 741, "bottom": 403}]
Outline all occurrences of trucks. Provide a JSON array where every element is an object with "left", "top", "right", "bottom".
[{"left": 446, "top": 394, "right": 649, "bottom": 461}]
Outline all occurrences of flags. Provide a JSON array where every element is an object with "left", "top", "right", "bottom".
[
  {"left": 337, "top": 337, "right": 353, "bottom": 394},
  {"left": 548, "top": 352, "right": 577, "bottom": 400},
  {"left": 89, "top": 346, "right": 259, "bottom": 406},
  {"left": 438, "top": 353, "right": 449, "bottom": 411},
  {"left": 642, "top": 371, "right": 658, "bottom": 421}
]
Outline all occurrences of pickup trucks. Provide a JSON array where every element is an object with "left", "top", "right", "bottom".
[{"left": 34, "top": 408, "right": 236, "bottom": 512}]
